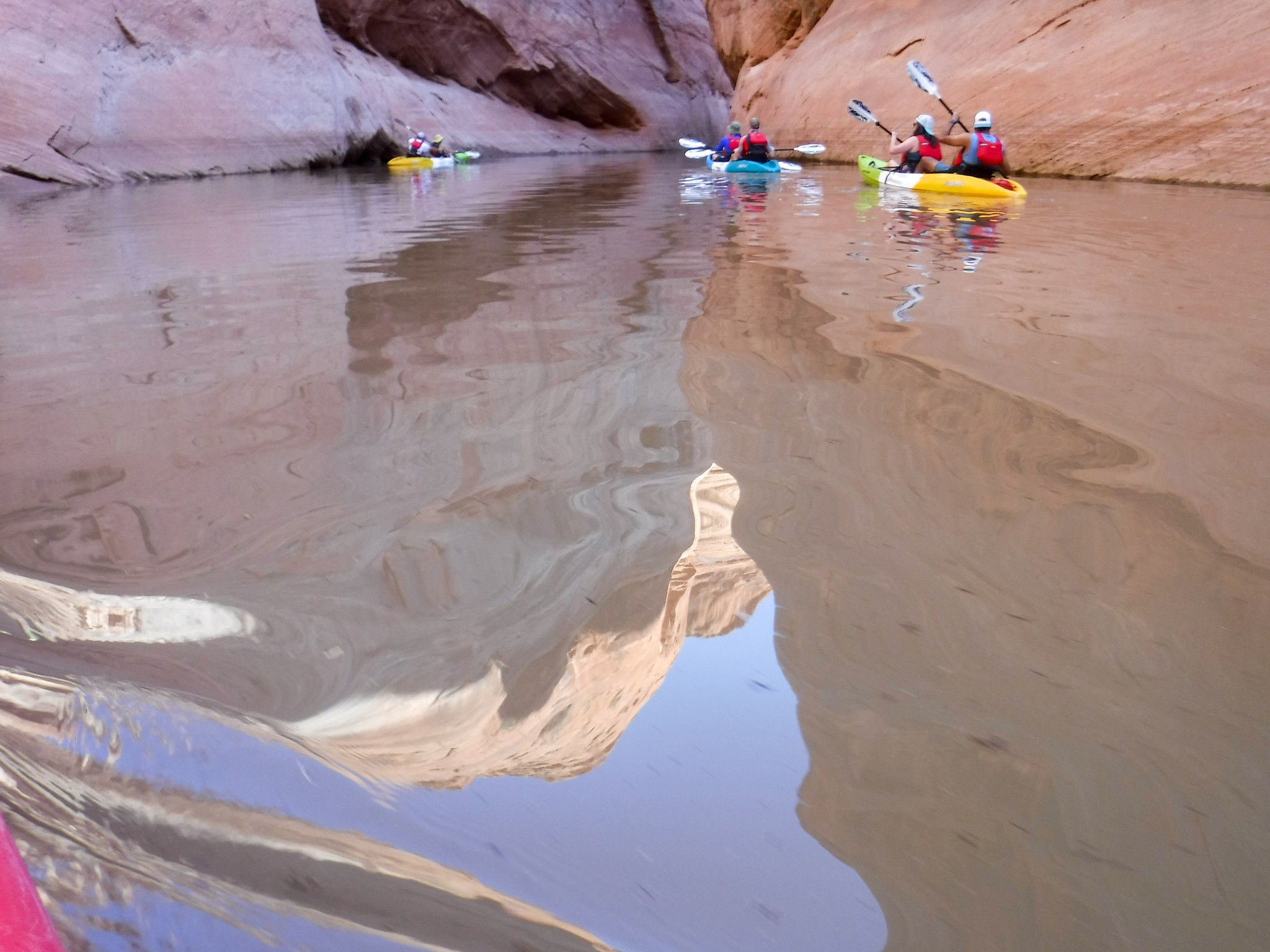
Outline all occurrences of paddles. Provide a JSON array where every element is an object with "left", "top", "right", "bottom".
[
  {"left": 449, "top": 150, "right": 480, "bottom": 159},
  {"left": 394, "top": 118, "right": 461, "bottom": 163},
  {"left": 685, "top": 143, "right": 826, "bottom": 159},
  {"left": 847, "top": 99, "right": 903, "bottom": 144},
  {"left": 678, "top": 137, "right": 802, "bottom": 170},
  {"left": 906, "top": 59, "right": 1009, "bottom": 180}
]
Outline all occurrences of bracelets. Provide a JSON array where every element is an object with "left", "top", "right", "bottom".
[{"left": 949, "top": 119, "right": 956, "bottom": 124}]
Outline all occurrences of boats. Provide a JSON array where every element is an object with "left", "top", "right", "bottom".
[
  {"left": 706, "top": 153, "right": 782, "bottom": 173},
  {"left": 388, "top": 150, "right": 456, "bottom": 168},
  {"left": 858, "top": 154, "right": 1027, "bottom": 199}
]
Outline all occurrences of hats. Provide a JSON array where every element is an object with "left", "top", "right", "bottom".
[
  {"left": 973, "top": 111, "right": 992, "bottom": 128},
  {"left": 432, "top": 134, "right": 444, "bottom": 143},
  {"left": 727, "top": 121, "right": 742, "bottom": 134},
  {"left": 749, "top": 117, "right": 760, "bottom": 129},
  {"left": 418, "top": 132, "right": 424, "bottom": 139},
  {"left": 916, "top": 114, "right": 935, "bottom": 136}
]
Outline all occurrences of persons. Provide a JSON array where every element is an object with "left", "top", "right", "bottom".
[
  {"left": 406, "top": 131, "right": 450, "bottom": 158},
  {"left": 711, "top": 117, "right": 774, "bottom": 164},
  {"left": 869, "top": 114, "right": 942, "bottom": 174},
  {"left": 913, "top": 111, "right": 1004, "bottom": 178}
]
